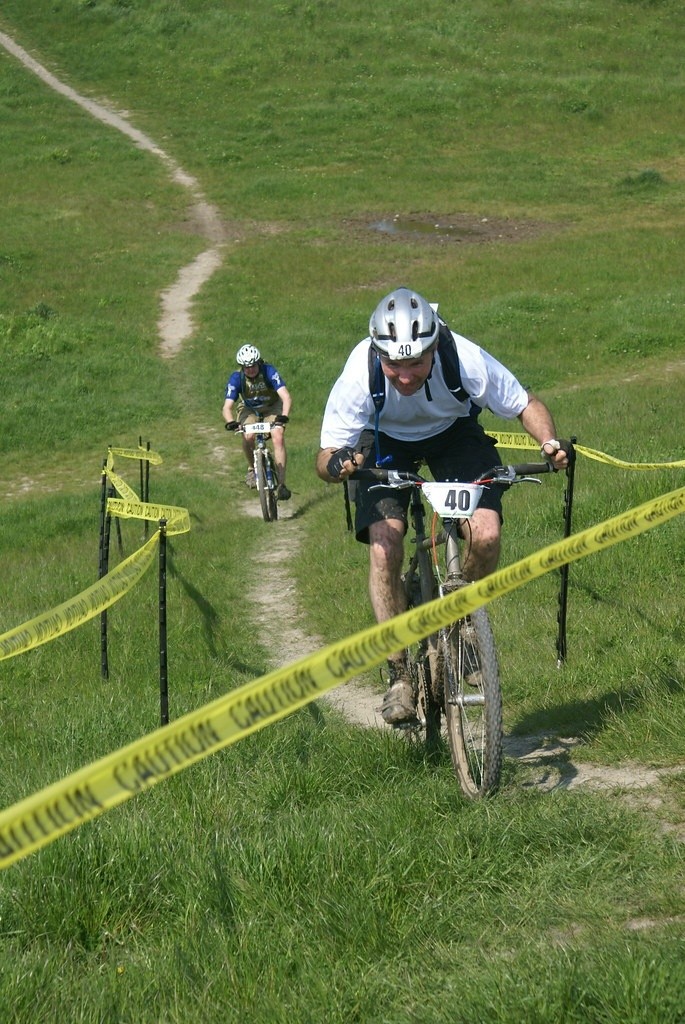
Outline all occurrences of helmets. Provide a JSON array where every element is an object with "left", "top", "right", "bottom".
[
  {"left": 368, "top": 288, "right": 439, "bottom": 360},
  {"left": 237, "top": 344, "right": 260, "bottom": 367}
]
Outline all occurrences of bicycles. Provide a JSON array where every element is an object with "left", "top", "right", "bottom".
[
  {"left": 225, "top": 415, "right": 289, "bottom": 522},
  {"left": 341, "top": 456, "right": 548, "bottom": 804}
]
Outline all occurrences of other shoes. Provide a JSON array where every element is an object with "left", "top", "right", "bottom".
[
  {"left": 278, "top": 485, "right": 291, "bottom": 499},
  {"left": 379, "top": 667, "right": 415, "bottom": 725},
  {"left": 245, "top": 466, "right": 256, "bottom": 488},
  {"left": 461, "top": 636, "right": 484, "bottom": 687}
]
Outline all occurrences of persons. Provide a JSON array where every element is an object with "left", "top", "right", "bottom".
[
  {"left": 222, "top": 343, "right": 292, "bottom": 499},
  {"left": 316, "top": 288, "right": 577, "bottom": 726}
]
